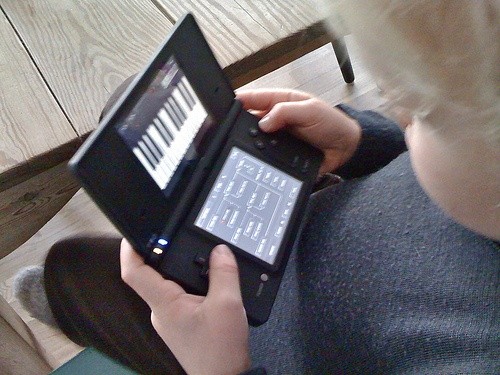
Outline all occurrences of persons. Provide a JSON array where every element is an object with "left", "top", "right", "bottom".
[{"left": 16, "top": 0, "right": 498, "bottom": 375}]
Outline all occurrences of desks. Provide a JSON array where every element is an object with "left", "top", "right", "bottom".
[{"left": 0, "top": 0, "right": 358, "bottom": 198}]
